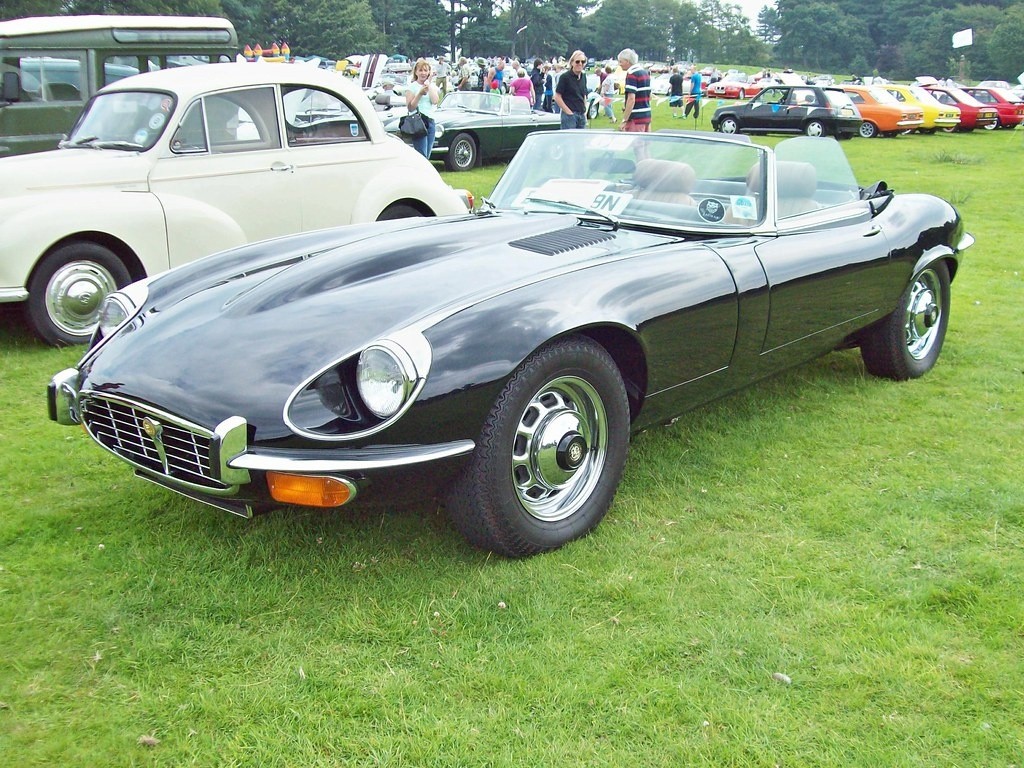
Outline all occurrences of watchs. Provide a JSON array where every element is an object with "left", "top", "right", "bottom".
[{"left": 622, "top": 119, "right": 627, "bottom": 124}]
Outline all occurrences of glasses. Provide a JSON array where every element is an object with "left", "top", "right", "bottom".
[{"left": 572, "top": 60, "right": 586, "bottom": 64}]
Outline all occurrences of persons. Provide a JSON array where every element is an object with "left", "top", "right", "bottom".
[
  {"left": 553, "top": 49, "right": 589, "bottom": 179},
  {"left": 710, "top": 66, "right": 718, "bottom": 84},
  {"left": 761, "top": 66, "right": 954, "bottom": 87},
  {"left": 669, "top": 68, "right": 686, "bottom": 117},
  {"left": 456, "top": 55, "right": 617, "bottom": 130},
  {"left": 432, "top": 57, "right": 451, "bottom": 98},
  {"left": 617, "top": 47, "right": 652, "bottom": 162},
  {"left": 405, "top": 57, "right": 440, "bottom": 162},
  {"left": 685, "top": 66, "right": 701, "bottom": 118}
]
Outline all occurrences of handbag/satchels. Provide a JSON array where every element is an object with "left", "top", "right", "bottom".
[{"left": 399, "top": 102, "right": 428, "bottom": 137}]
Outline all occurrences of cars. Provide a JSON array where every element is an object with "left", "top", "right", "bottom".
[
  {"left": 0, "top": 15, "right": 240, "bottom": 169},
  {"left": 44, "top": 121, "right": 974, "bottom": 564},
  {"left": 979, "top": 85, "right": 1024, "bottom": 133},
  {"left": 840, "top": 86, "right": 923, "bottom": 138},
  {"left": 1012, "top": 84, "right": 1024, "bottom": 101},
  {"left": 885, "top": 85, "right": 960, "bottom": 133},
  {"left": 927, "top": 83, "right": 998, "bottom": 133},
  {"left": 383, "top": 89, "right": 561, "bottom": 171},
  {"left": 974, "top": 80, "right": 1011, "bottom": 90},
  {"left": 711, "top": 88, "right": 864, "bottom": 141},
  {"left": 706, "top": 76, "right": 764, "bottom": 100},
  {"left": 0, "top": 62, "right": 475, "bottom": 347},
  {"left": 13, "top": 55, "right": 140, "bottom": 102}
]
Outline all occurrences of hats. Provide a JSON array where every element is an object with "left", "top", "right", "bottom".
[{"left": 491, "top": 80, "right": 499, "bottom": 89}]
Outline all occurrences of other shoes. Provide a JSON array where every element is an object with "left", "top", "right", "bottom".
[
  {"left": 673, "top": 115, "right": 677, "bottom": 118},
  {"left": 679, "top": 115, "right": 686, "bottom": 119}
]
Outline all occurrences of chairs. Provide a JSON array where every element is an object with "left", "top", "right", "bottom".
[
  {"left": 722, "top": 160, "right": 823, "bottom": 225},
  {"left": 625, "top": 158, "right": 700, "bottom": 207}
]
